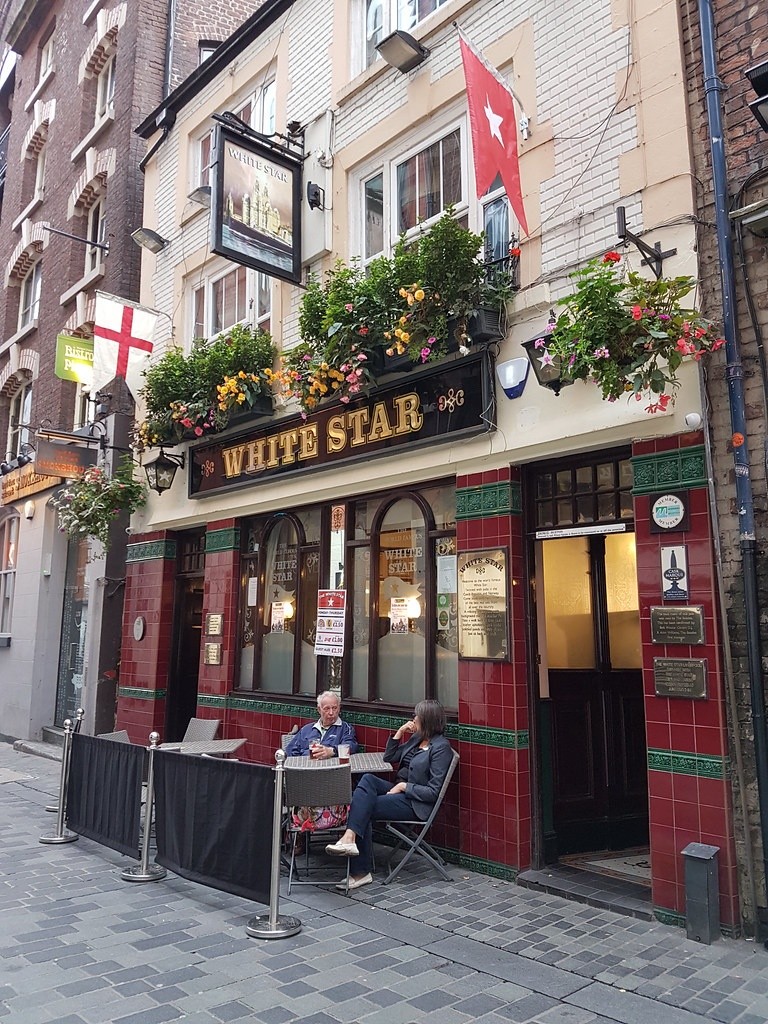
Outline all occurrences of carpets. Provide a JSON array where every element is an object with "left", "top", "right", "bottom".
[{"left": 562, "top": 848, "right": 650, "bottom": 887}]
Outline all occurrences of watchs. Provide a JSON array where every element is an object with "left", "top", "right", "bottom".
[{"left": 330, "top": 747, "right": 336, "bottom": 757}]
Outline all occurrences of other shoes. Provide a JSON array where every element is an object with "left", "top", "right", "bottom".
[
  {"left": 290, "top": 837, "right": 306, "bottom": 854},
  {"left": 325, "top": 841, "right": 359, "bottom": 856},
  {"left": 336, "top": 872, "right": 373, "bottom": 889}
]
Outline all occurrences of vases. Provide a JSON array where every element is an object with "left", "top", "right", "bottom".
[
  {"left": 147, "top": 396, "right": 273, "bottom": 448},
  {"left": 588, "top": 331, "right": 647, "bottom": 367},
  {"left": 294, "top": 307, "right": 506, "bottom": 395}
]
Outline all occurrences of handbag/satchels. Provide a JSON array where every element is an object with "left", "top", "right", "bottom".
[{"left": 292, "top": 804, "right": 351, "bottom": 829}]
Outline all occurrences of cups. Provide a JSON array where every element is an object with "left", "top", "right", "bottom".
[
  {"left": 338, "top": 744, "right": 350, "bottom": 766},
  {"left": 309, "top": 738, "right": 320, "bottom": 761}
]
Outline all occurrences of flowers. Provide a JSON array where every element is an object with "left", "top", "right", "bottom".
[
  {"left": 536, "top": 245, "right": 728, "bottom": 415},
  {"left": 272, "top": 205, "right": 520, "bottom": 420},
  {"left": 51, "top": 453, "right": 149, "bottom": 549},
  {"left": 123, "top": 322, "right": 278, "bottom": 453}
]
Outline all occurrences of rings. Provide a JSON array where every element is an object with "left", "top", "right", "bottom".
[
  {"left": 410, "top": 724, "right": 411, "bottom": 726},
  {"left": 322, "top": 752, "right": 324, "bottom": 755}
]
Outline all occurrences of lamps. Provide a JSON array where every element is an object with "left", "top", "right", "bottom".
[
  {"left": 142, "top": 445, "right": 185, "bottom": 496},
  {"left": 306, "top": 180, "right": 325, "bottom": 211},
  {"left": 17, "top": 443, "right": 35, "bottom": 468},
  {"left": 520, "top": 310, "right": 589, "bottom": 396},
  {"left": 185, "top": 186, "right": 211, "bottom": 207},
  {"left": 0, "top": 452, "right": 17, "bottom": 476},
  {"left": 24, "top": 500, "right": 35, "bottom": 519},
  {"left": 374, "top": 28, "right": 431, "bottom": 75},
  {"left": 129, "top": 227, "right": 170, "bottom": 254}
]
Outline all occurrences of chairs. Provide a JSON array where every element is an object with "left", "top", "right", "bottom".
[
  {"left": 182, "top": 717, "right": 221, "bottom": 741},
  {"left": 283, "top": 763, "right": 352, "bottom": 896},
  {"left": 376, "top": 748, "right": 460, "bottom": 886},
  {"left": 97, "top": 730, "right": 131, "bottom": 743}
]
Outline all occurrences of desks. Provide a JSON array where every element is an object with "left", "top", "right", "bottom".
[
  {"left": 158, "top": 738, "right": 248, "bottom": 759},
  {"left": 284, "top": 752, "right": 394, "bottom": 874}
]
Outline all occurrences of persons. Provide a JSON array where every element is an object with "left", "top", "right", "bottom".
[
  {"left": 325, "top": 700, "right": 453, "bottom": 889},
  {"left": 282, "top": 690, "right": 358, "bottom": 856}
]
